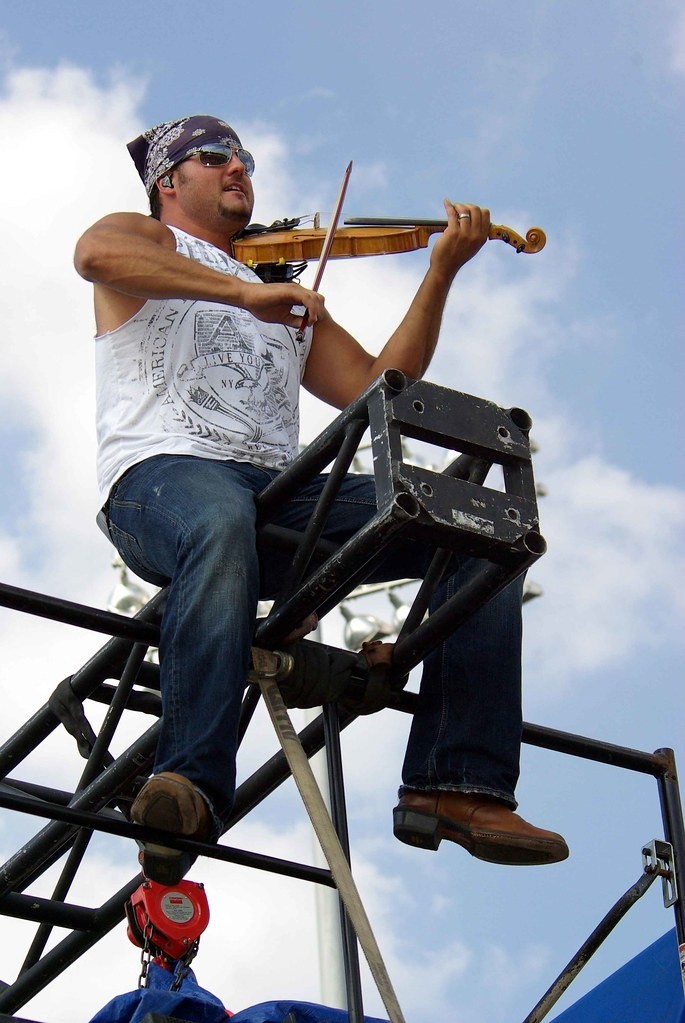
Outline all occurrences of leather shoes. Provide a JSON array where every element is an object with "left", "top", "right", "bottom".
[
  {"left": 390, "top": 790, "right": 570, "bottom": 866},
  {"left": 128, "top": 774, "right": 209, "bottom": 887}
]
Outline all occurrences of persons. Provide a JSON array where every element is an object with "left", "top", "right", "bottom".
[{"left": 75, "top": 115, "right": 570, "bottom": 889}]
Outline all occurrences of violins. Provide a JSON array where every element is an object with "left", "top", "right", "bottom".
[{"left": 230, "top": 211, "right": 547, "bottom": 270}]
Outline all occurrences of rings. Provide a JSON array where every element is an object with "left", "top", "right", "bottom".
[{"left": 459, "top": 213, "right": 471, "bottom": 219}]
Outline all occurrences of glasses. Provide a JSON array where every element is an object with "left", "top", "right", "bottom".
[{"left": 178, "top": 143, "right": 255, "bottom": 178}]
